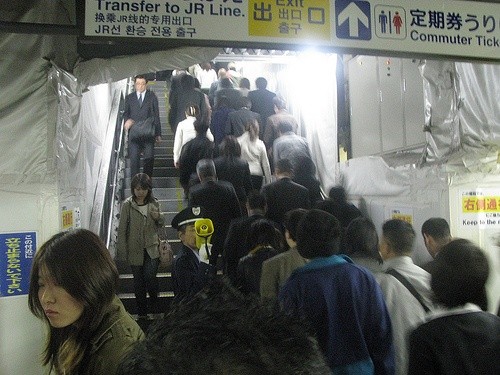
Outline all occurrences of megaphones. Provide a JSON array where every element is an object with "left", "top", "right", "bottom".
[{"left": 194, "top": 219, "right": 214, "bottom": 248}]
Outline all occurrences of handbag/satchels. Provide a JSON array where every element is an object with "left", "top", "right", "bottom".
[
  {"left": 158, "top": 240, "right": 173, "bottom": 265},
  {"left": 128, "top": 117, "right": 155, "bottom": 141}
]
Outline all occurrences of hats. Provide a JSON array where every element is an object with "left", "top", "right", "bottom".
[{"left": 171, "top": 206, "right": 206, "bottom": 229}]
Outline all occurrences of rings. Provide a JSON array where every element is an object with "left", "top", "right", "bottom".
[{"left": 160, "top": 140, "right": 161, "bottom": 141}]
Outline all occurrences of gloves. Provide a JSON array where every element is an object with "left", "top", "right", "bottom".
[{"left": 199, "top": 243, "right": 212, "bottom": 264}]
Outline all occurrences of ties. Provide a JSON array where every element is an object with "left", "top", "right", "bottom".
[{"left": 138, "top": 94, "right": 142, "bottom": 109}]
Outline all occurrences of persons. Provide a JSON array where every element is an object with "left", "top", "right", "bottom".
[
  {"left": 170, "top": 205, "right": 217, "bottom": 304},
  {"left": 117, "top": 172, "right": 167, "bottom": 319},
  {"left": 374, "top": 219, "right": 435, "bottom": 375},
  {"left": 419, "top": 218, "right": 487, "bottom": 312},
  {"left": 116, "top": 281, "right": 333, "bottom": 375},
  {"left": 166, "top": 61, "right": 385, "bottom": 306},
  {"left": 27, "top": 228, "right": 146, "bottom": 375},
  {"left": 123, "top": 76, "right": 162, "bottom": 181},
  {"left": 277, "top": 209, "right": 397, "bottom": 375},
  {"left": 409, "top": 239, "right": 500, "bottom": 375}
]
